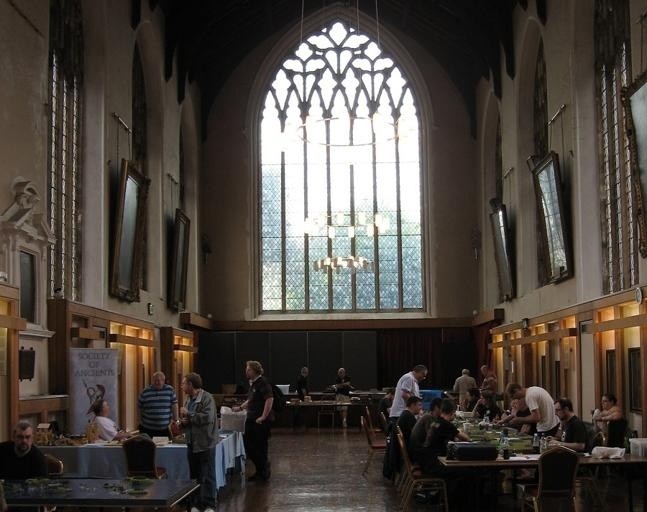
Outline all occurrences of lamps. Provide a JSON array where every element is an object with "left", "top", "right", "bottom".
[{"left": 295, "top": 0, "right": 402, "bottom": 274}]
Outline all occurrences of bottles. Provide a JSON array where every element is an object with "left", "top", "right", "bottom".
[
  {"left": 539, "top": 432, "right": 547, "bottom": 454},
  {"left": 503, "top": 439, "right": 509, "bottom": 459},
  {"left": 532, "top": 432, "right": 539, "bottom": 452}
]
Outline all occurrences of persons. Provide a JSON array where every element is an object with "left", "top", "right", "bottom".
[
  {"left": 231, "top": 360, "right": 274, "bottom": 483},
  {"left": 1, "top": 418, "right": 46, "bottom": 480},
  {"left": 335, "top": 367, "right": 352, "bottom": 427},
  {"left": 87, "top": 399, "right": 132, "bottom": 442},
  {"left": 135, "top": 371, "right": 179, "bottom": 441},
  {"left": 295, "top": 366, "right": 311, "bottom": 402},
  {"left": 180, "top": 372, "right": 220, "bottom": 512},
  {"left": 588, "top": 392, "right": 623, "bottom": 447},
  {"left": 378, "top": 364, "right": 588, "bottom": 512}
]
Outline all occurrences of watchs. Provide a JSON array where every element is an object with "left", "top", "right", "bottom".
[{"left": 175, "top": 419, "right": 180, "bottom": 423}]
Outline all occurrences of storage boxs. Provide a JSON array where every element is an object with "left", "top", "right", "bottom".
[{"left": 628, "top": 437, "right": 647, "bottom": 457}]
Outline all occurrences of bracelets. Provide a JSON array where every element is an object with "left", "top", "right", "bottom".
[{"left": 239, "top": 406, "right": 243, "bottom": 411}]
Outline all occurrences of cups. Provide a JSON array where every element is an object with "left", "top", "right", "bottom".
[{"left": 464, "top": 422, "right": 471, "bottom": 433}]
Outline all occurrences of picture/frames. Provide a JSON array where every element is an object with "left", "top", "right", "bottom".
[
  {"left": 489, "top": 204, "right": 517, "bottom": 304},
  {"left": 108, "top": 158, "right": 152, "bottom": 303},
  {"left": 167, "top": 207, "right": 191, "bottom": 313},
  {"left": 531, "top": 150, "right": 575, "bottom": 289},
  {"left": 619, "top": 69, "right": 647, "bottom": 260}
]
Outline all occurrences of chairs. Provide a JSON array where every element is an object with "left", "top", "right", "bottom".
[
  {"left": 575, "top": 431, "right": 609, "bottom": 512},
  {"left": 519, "top": 446, "right": 580, "bottom": 512},
  {"left": 47, "top": 455, "right": 65, "bottom": 512},
  {"left": 361, "top": 405, "right": 449, "bottom": 512},
  {"left": 120, "top": 435, "right": 168, "bottom": 511}
]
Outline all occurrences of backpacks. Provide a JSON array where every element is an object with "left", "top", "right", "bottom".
[{"left": 272, "top": 386, "right": 286, "bottom": 411}]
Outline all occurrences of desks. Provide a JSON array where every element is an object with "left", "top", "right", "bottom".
[{"left": 419, "top": 389, "right": 443, "bottom": 410}]
